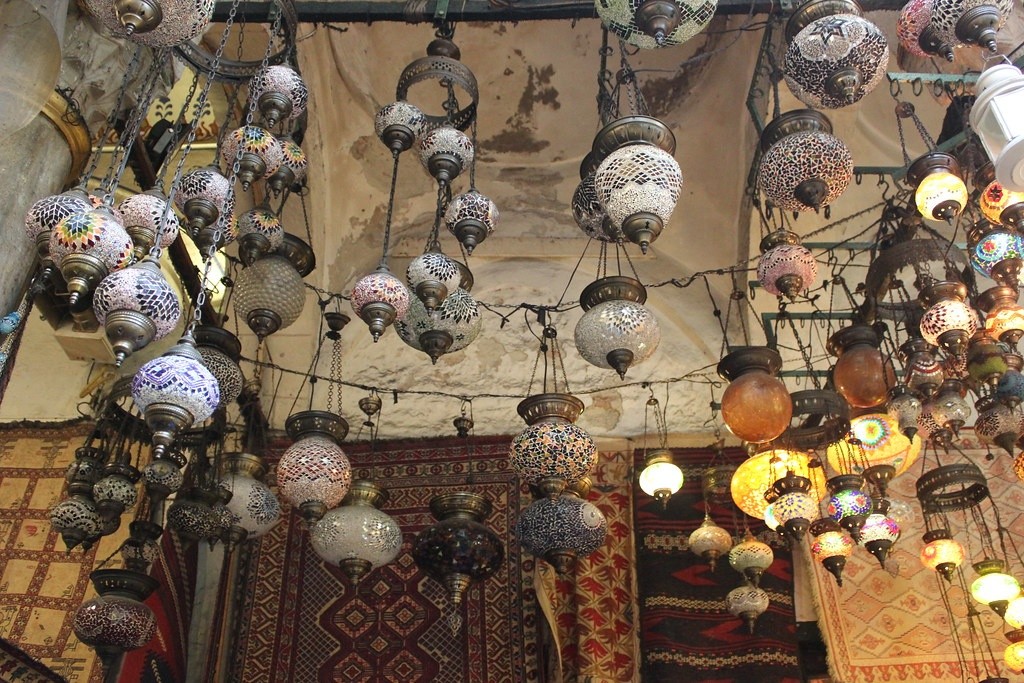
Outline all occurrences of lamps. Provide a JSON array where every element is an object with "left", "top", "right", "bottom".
[{"left": 0, "top": 0, "right": 1024, "bottom": 683}]
